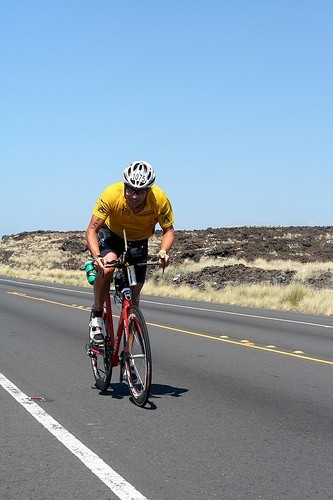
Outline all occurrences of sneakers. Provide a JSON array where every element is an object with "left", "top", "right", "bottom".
[
  {"left": 129, "top": 355, "right": 137, "bottom": 383},
  {"left": 89, "top": 311, "right": 105, "bottom": 342}
]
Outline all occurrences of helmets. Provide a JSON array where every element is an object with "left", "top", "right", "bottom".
[{"left": 122, "top": 160, "right": 156, "bottom": 191}]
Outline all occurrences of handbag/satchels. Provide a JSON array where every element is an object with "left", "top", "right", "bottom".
[{"left": 97, "top": 224, "right": 115, "bottom": 251}]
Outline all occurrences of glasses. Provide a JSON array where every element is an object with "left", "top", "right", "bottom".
[{"left": 126, "top": 186, "right": 150, "bottom": 196}]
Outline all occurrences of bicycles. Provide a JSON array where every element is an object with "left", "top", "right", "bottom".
[{"left": 85, "top": 242, "right": 166, "bottom": 407}]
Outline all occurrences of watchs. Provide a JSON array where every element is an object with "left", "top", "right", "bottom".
[{"left": 91, "top": 255, "right": 103, "bottom": 267}]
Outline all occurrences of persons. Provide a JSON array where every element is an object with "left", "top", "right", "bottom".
[{"left": 88, "top": 161, "right": 176, "bottom": 384}]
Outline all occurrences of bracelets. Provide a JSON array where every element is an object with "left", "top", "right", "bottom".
[{"left": 159, "top": 250, "right": 167, "bottom": 254}]
